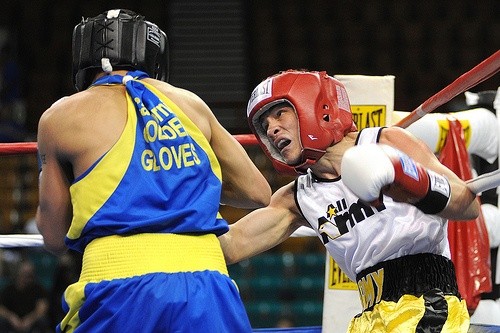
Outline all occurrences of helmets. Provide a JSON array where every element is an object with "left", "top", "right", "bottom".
[
  {"left": 247, "top": 69, "right": 356, "bottom": 178},
  {"left": 72, "top": 9, "right": 170, "bottom": 91}
]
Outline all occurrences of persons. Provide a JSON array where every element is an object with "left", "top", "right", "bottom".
[
  {"left": 218, "top": 68, "right": 481, "bottom": 333},
  {"left": 36, "top": 8, "right": 272, "bottom": 333},
  {"left": 0, "top": 260, "right": 49, "bottom": 333}
]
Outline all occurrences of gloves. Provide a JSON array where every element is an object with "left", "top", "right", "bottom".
[{"left": 342, "top": 147, "right": 451, "bottom": 216}]
